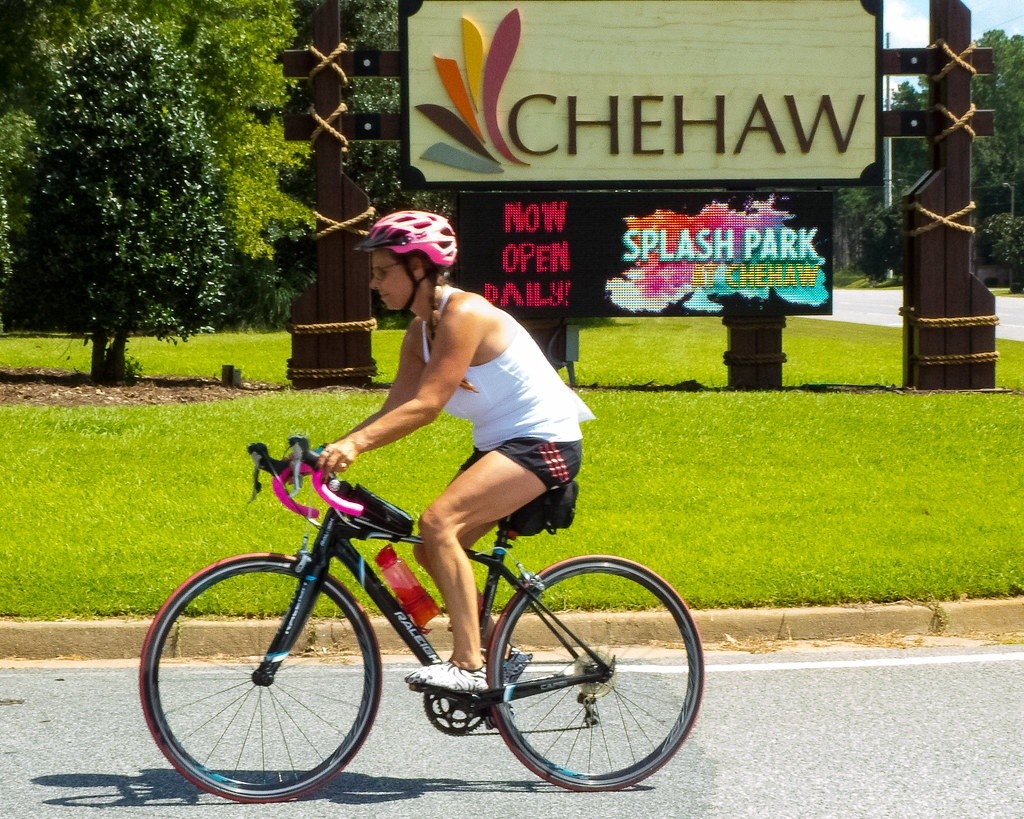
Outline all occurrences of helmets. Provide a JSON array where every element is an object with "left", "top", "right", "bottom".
[{"left": 352, "top": 210, "right": 457, "bottom": 266}]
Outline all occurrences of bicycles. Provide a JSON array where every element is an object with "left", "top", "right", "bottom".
[{"left": 137, "top": 434, "right": 707, "bottom": 805}]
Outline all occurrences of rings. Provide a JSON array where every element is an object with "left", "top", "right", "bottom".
[
  {"left": 339, "top": 463, "right": 347, "bottom": 467},
  {"left": 321, "top": 451, "right": 328, "bottom": 456}
]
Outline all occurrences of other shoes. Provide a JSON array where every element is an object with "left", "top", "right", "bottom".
[
  {"left": 405, "top": 661, "right": 489, "bottom": 692},
  {"left": 502, "top": 647, "right": 533, "bottom": 683}
]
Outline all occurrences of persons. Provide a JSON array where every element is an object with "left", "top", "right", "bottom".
[{"left": 318, "top": 210, "right": 598, "bottom": 694}]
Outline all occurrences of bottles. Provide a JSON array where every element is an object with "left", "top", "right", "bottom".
[{"left": 375, "top": 545, "right": 440, "bottom": 624}]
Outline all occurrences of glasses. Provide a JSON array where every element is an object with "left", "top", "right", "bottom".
[{"left": 372, "top": 262, "right": 398, "bottom": 280}]
